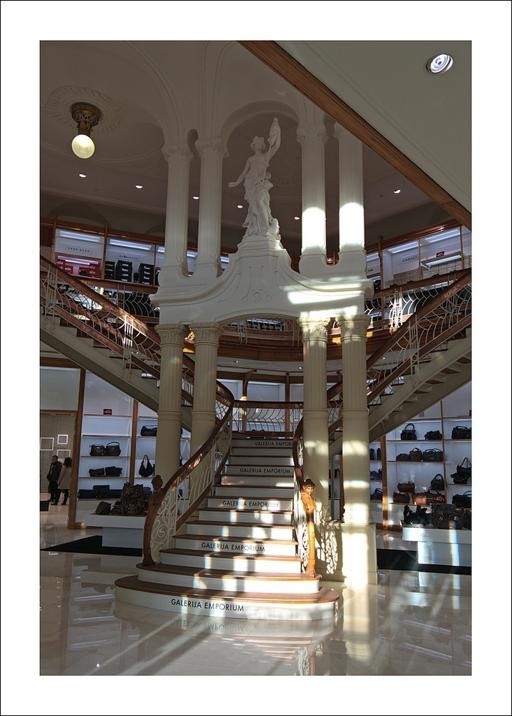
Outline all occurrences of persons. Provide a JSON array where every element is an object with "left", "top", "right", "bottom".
[
  {"left": 47, "top": 454, "right": 63, "bottom": 502},
  {"left": 51, "top": 456, "right": 73, "bottom": 505},
  {"left": 228, "top": 117, "right": 283, "bottom": 225}
]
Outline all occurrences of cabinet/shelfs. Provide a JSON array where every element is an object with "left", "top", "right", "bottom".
[
  {"left": 368, "top": 378, "right": 470, "bottom": 532},
  {"left": 215, "top": 379, "right": 340, "bottom": 431},
  {"left": 366, "top": 255, "right": 470, "bottom": 318},
  {"left": 76, "top": 369, "right": 192, "bottom": 527},
  {"left": 55, "top": 257, "right": 161, "bottom": 320},
  {"left": 244, "top": 319, "right": 285, "bottom": 332}
]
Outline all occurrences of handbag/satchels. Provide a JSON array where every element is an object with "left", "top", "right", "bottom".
[
  {"left": 370, "top": 423, "right": 471, "bottom": 531},
  {"left": 78, "top": 425, "right": 157, "bottom": 515}
]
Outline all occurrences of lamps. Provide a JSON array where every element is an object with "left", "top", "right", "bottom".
[{"left": 70, "top": 102, "right": 102, "bottom": 160}]
